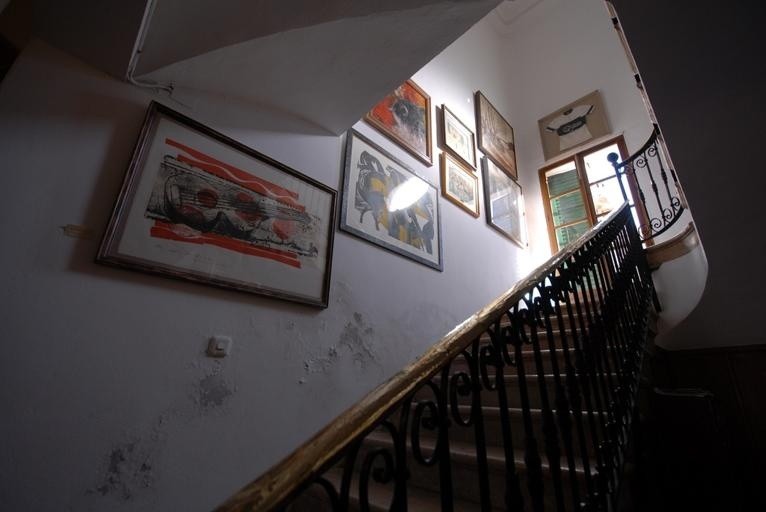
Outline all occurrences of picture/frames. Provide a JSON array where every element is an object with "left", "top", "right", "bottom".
[
  {"left": 480, "top": 154, "right": 527, "bottom": 251},
  {"left": 472, "top": 88, "right": 521, "bottom": 181},
  {"left": 438, "top": 149, "right": 483, "bottom": 220},
  {"left": 93, "top": 92, "right": 340, "bottom": 311},
  {"left": 439, "top": 101, "right": 480, "bottom": 174},
  {"left": 337, "top": 122, "right": 448, "bottom": 277},
  {"left": 364, "top": 78, "right": 433, "bottom": 168}
]
